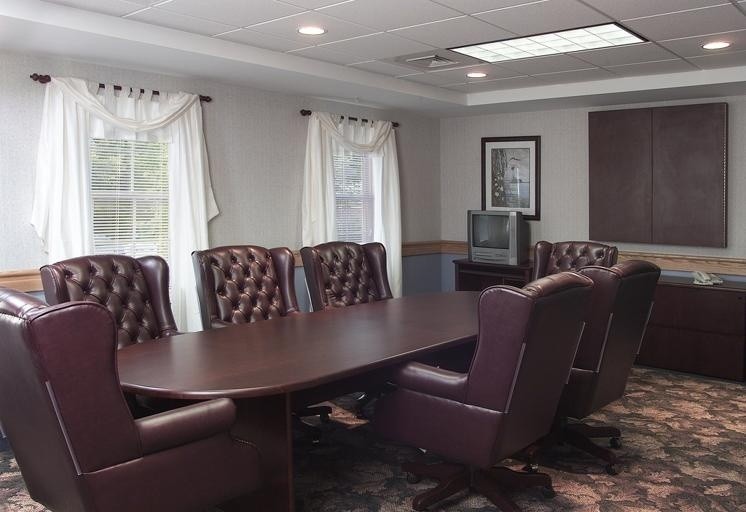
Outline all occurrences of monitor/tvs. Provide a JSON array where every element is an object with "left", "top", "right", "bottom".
[{"left": 467, "top": 210, "right": 530, "bottom": 266}]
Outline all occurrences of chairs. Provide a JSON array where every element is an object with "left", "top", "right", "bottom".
[
  {"left": 0, "top": 284, "right": 298, "bottom": 512},
  {"left": 532, "top": 241, "right": 618, "bottom": 280},
  {"left": 39, "top": 254, "right": 183, "bottom": 350},
  {"left": 525, "top": 260, "right": 660, "bottom": 476},
  {"left": 192, "top": 245, "right": 332, "bottom": 424},
  {"left": 300, "top": 241, "right": 393, "bottom": 312},
  {"left": 387, "top": 271, "right": 594, "bottom": 512}
]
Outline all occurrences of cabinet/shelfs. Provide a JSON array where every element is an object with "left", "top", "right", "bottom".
[{"left": 588, "top": 102, "right": 728, "bottom": 248}]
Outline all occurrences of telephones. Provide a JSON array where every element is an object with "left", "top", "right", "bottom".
[{"left": 692, "top": 271, "right": 723, "bottom": 284}]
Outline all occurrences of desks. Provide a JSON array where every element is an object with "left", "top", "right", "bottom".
[
  {"left": 640, "top": 270, "right": 746, "bottom": 382},
  {"left": 116, "top": 291, "right": 481, "bottom": 509},
  {"left": 453, "top": 258, "right": 534, "bottom": 293}
]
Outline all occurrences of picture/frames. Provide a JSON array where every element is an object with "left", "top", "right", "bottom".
[{"left": 481, "top": 136, "right": 541, "bottom": 219}]
